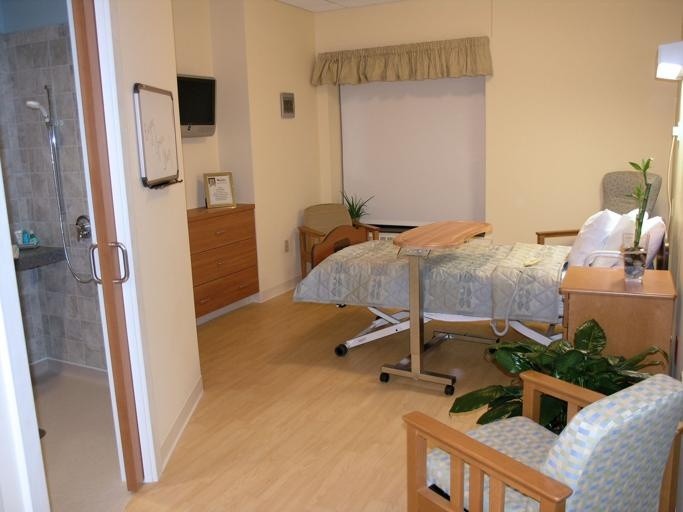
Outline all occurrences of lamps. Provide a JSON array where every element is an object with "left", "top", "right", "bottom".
[{"left": 656, "top": 40, "right": 683, "bottom": 79}]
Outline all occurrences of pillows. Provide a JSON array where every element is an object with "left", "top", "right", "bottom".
[
  {"left": 567, "top": 209, "right": 621, "bottom": 266},
  {"left": 593, "top": 206, "right": 648, "bottom": 267}
]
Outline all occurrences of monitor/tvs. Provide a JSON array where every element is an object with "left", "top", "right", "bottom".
[{"left": 177, "top": 73, "right": 217, "bottom": 138}]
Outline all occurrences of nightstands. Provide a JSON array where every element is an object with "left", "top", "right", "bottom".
[{"left": 558, "top": 266, "right": 677, "bottom": 378}]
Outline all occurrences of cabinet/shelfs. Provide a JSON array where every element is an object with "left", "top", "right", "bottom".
[{"left": 188, "top": 204, "right": 259, "bottom": 318}]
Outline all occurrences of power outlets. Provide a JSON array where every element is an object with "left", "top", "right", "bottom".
[{"left": 677, "top": 121, "right": 680, "bottom": 141}]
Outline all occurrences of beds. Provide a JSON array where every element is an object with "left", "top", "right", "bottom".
[{"left": 293, "top": 216, "right": 666, "bottom": 357}]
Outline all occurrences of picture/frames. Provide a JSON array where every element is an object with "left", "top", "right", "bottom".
[{"left": 203, "top": 171, "right": 236, "bottom": 209}]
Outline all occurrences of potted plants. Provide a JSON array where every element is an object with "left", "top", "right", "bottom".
[
  {"left": 339, "top": 189, "right": 375, "bottom": 226},
  {"left": 620, "top": 156, "right": 652, "bottom": 285}
]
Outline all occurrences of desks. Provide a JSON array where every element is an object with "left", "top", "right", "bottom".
[{"left": 378, "top": 220, "right": 493, "bottom": 394}]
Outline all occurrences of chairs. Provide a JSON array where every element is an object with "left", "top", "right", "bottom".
[
  {"left": 297, "top": 203, "right": 380, "bottom": 280},
  {"left": 401, "top": 370, "right": 683, "bottom": 512},
  {"left": 536, "top": 172, "right": 661, "bottom": 247}
]
